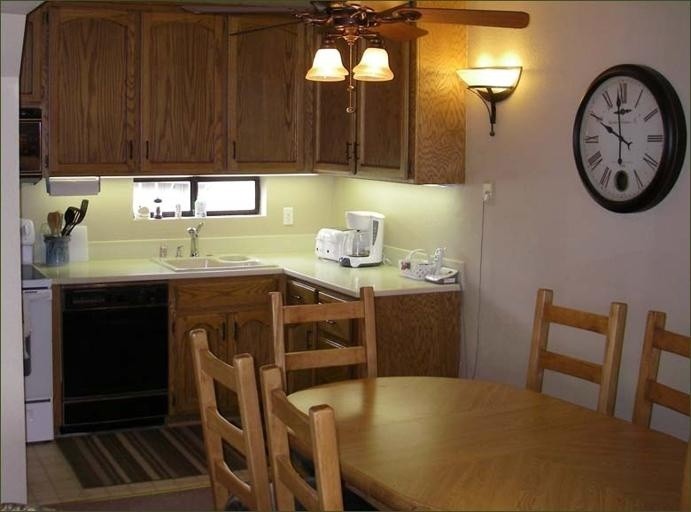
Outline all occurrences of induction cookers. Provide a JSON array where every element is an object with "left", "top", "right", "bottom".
[{"left": 21, "top": 263, "right": 47, "bottom": 281}]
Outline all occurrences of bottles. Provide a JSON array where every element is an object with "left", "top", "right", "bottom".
[{"left": 195, "top": 196, "right": 207, "bottom": 218}]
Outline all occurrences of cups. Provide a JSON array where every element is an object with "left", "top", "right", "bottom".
[{"left": 43, "top": 234, "right": 71, "bottom": 265}]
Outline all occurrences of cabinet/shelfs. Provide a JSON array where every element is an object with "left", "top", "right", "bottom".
[
  {"left": 313, "top": 0, "right": 467, "bottom": 186},
  {"left": 229, "top": 0, "right": 313, "bottom": 176},
  {"left": 18, "top": 1, "right": 229, "bottom": 175},
  {"left": 173, "top": 277, "right": 283, "bottom": 418},
  {"left": 284, "top": 277, "right": 359, "bottom": 394}
]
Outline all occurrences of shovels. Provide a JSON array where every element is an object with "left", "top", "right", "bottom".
[{"left": 47, "top": 200, "right": 88, "bottom": 236}]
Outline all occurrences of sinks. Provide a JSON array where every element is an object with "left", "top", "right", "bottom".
[{"left": 152, "top": 253, "right": 279, "bottom": 271}]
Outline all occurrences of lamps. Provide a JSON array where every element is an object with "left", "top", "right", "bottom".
[
  {"left": 304, "top": 2, "right": 395, "bottom": 115},
  {"left": 453, "top": 66, "right": 520, "bottom": 138}
]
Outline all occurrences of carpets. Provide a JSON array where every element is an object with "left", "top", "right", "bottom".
[{"left": 53, "top": 418, "right": 260, "bottom": 489}]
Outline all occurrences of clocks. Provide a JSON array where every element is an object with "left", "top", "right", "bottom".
[{"left": 573, "top": 63, "right": 689, "bottom": 216}]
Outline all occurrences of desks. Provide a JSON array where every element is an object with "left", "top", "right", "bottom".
[{"left": 284, "top": 376, "right": 691, "bottom": 512}]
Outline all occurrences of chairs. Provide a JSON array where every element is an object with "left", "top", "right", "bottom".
[
  {"left": 258, "top": 365, "right": 344, "bottom": 512},
  {"left": 267, "top": 286, "right": 378, "bottom": 381},
  {"left": 524, "top": 287, "right": 628, "bottom": 421},
  {"left": 630, "top": 308, "right": 689, "bottom": 426},
  {"left": 189, "top": 328, "right": 270, "bottom": 510}
]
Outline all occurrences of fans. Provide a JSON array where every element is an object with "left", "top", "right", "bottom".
[{"left": 180, "top": 0, "right": 532, "bottom": 37}]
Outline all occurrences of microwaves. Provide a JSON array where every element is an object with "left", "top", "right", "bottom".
[{"left": 19, "top": 107, "right": 45, "bottom": 177}]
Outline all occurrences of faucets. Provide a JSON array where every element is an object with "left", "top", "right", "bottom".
[{"left": 187, "top": 223, "right": 204, "bottom": 257}]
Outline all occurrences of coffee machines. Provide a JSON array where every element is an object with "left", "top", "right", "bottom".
[{"left": 339, "top": 210, "right": 386, "bottom": 269}]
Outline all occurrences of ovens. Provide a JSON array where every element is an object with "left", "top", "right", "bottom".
[{"left": 22, "top": 286, "right": 55, "bottom": 445}]
